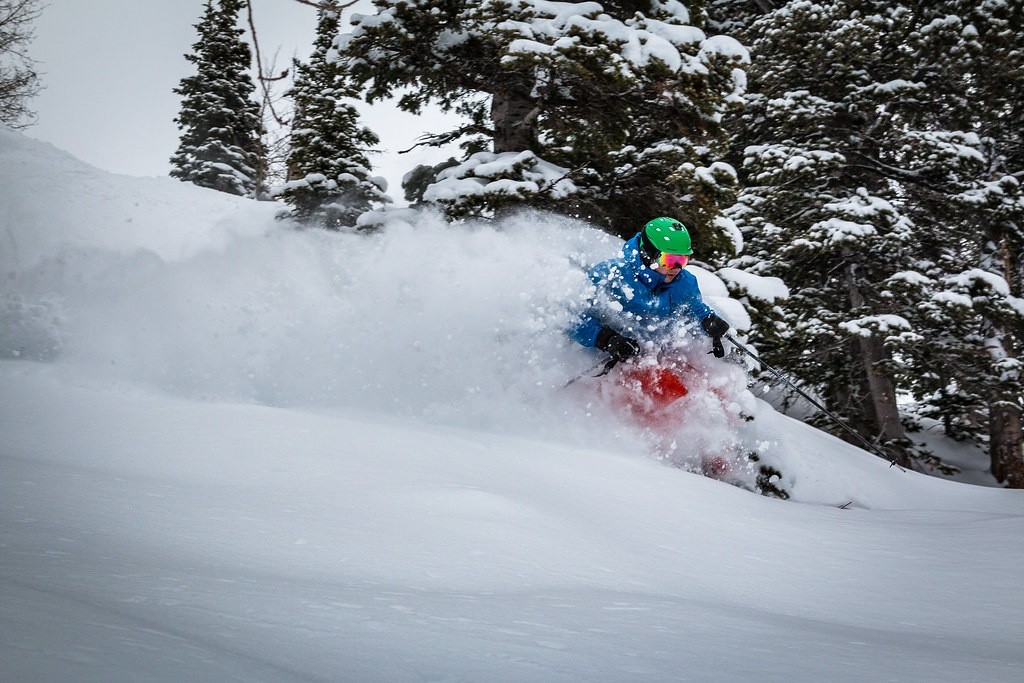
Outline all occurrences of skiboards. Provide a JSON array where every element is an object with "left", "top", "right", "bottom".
[{"left": 838, "top": 501, "right": 853, "bottom": 509}]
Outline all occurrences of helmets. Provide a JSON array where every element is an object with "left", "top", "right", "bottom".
[{"left": 639, "top": 217, "right": 694, "bottom": 270}]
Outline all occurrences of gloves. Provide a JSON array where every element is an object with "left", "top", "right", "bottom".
[
  {"left": 700, "top": 312, "right": 730, "bottom": 340},
  {"left": 594, "top": 325, "right": 640, "bottom": 363}
]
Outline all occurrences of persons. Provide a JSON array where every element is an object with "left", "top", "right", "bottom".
[{"left": 564, "top": 216, "right": 787, "bottom": 498}]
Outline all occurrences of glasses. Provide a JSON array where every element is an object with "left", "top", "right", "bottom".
[{"left": 656, "top": 252, "right": 689, "bottom": 270}]
